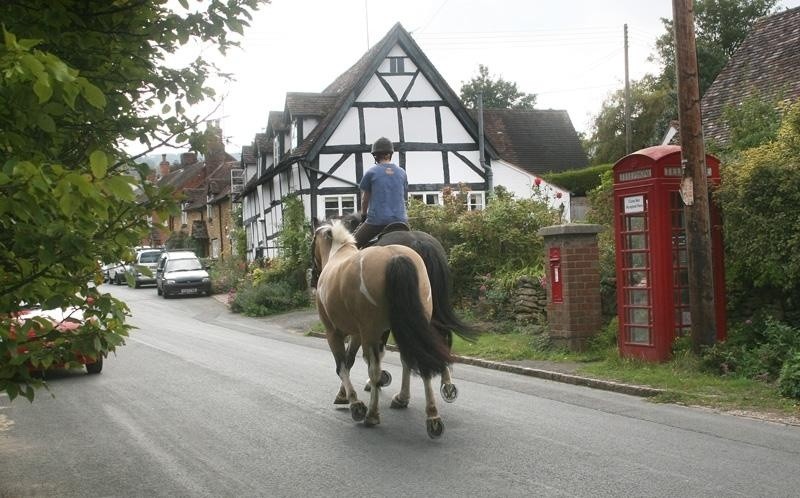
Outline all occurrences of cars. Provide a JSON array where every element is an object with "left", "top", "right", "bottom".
[
  {"left": 1, "top": 282, "right": 106, "bottom": 377},
  {"left": 90, "top": 238, "right": 164, "bottom": 288}
]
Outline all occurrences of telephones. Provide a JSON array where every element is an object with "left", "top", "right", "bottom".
[{"left": 672, "top": 233, "right": 688, "bottom": 272}]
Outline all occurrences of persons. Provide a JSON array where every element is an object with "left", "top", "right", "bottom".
[{"left": 351, "top": 137, "right": 409, "bottom": 249}]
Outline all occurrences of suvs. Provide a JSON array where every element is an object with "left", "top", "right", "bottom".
[{"left": 155, "top": 245, "right": 212, "bottom": 300}]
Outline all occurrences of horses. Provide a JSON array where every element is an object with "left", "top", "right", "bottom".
[
  {"left": 343, "top": 211, "right": 482, "bottom": 410},
  {"left": 306, "top": 215, "right": 450, "bottom": 443}
]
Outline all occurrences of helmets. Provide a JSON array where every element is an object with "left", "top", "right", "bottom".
[{"left": 371, "top": 136, "right": 394, "bottom": 154}]
